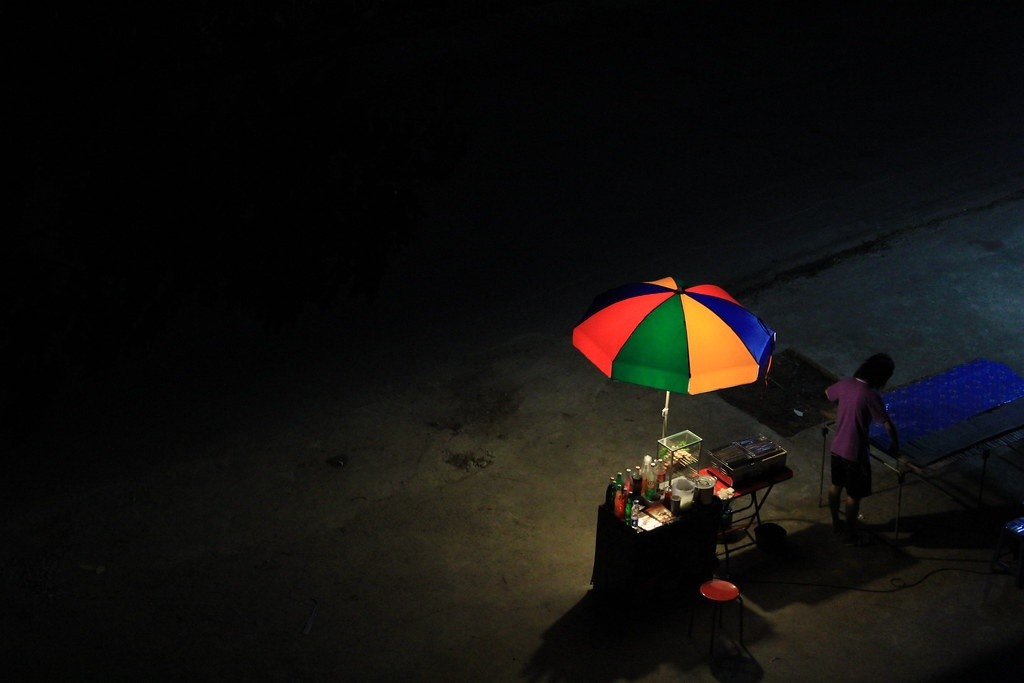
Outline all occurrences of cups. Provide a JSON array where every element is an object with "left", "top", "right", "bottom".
[{"left": 675, "top": 479, "right": 695, "bottom": 508}]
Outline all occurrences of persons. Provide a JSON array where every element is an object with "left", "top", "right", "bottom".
[{"left": 824, "top": 353, "right": 900, "bottom": 529}]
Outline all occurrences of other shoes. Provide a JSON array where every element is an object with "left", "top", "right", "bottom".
[
  {"left": 832, "top": 518, "right": 845, "bottom": 535},
  {"left": 843, "top": 532, "right": 873, "bottom": 546}
]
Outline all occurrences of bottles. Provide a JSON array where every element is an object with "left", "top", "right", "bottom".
[{"left": 606, "top": 455, "right": 673, "bottom": 529}]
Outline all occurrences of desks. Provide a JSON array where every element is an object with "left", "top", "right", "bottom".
[
  {"left": 591, "top": 494, "right": 725, "bottom": 612},
  {"left": 816, "top": 357, "right": 1023, "bottom": 561},
  {"left": 692, "top": 463, "right": 794, "bottom": 567}
]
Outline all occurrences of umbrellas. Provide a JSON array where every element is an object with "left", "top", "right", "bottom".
[{"left": 572, "top": 273, "right": 777, "bottom": 438}]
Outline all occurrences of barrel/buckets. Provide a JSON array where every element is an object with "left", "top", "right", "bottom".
[{"left": 694, "top": 476, "right": 716, "bottom": 503}]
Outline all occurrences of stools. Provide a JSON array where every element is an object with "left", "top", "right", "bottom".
[
  {"left": 989, "top": 515, "right": 1023, "bottom": 588},
  {"left": 684, "top": 579, "right": 744, "bottom": 658}
]
